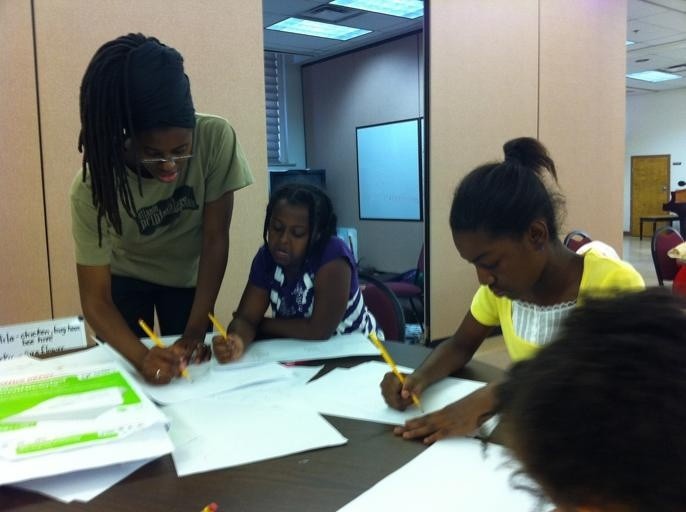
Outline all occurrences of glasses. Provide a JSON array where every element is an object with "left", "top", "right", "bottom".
[{"left": 139, "top": 153, "right": 193, "bottom": 167}]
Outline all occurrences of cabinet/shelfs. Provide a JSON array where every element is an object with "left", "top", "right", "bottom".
[{"left": 271, "top": 170, "right": 326, "bottom": 194}]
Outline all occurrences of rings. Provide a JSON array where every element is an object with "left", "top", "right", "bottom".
[{"left": 155, "top": 368, "right": 161, "bottom": 376}]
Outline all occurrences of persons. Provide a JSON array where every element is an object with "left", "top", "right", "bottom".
[
  {"left": 485, "top": 284, "right": 686, "bottom": 512},
  {"left": 68, "top": 30, "right": 256, "bottom": 386},
  {"left": 377, "top": 135, "right": 644, "bottom": 446},
  {"left": 209, "top": 181, "right": 387, "bottom": 367}
]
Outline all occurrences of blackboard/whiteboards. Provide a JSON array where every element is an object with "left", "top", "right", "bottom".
[{"left": 356, "top": 118, "right": 424, "bottom": 221}]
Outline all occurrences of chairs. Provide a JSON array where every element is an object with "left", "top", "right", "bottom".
[
  {"left": 564, "top": 227, "right": 686, "bottom": 299},
  {"left": 358, "top": 243, "right": 424, "bottom": 343}
]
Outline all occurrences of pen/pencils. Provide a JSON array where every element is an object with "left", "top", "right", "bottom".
[
  {"left": 369, "top": 333, "right": 424, "bottom": 413},
  {"left": 138, "top": 320, "right": 193, "bottom": 383},
  {"left": 208, "top": 313, "right": 226, "bottom": 338}
]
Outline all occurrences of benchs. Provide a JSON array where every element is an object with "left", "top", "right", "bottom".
[{"left": 639, "top": 215, "right": 680, "bottom": 241}]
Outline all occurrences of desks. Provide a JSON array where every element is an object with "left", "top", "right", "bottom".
[{"left": 0, "top": 337, "right": 511, "bottom": 512}]
